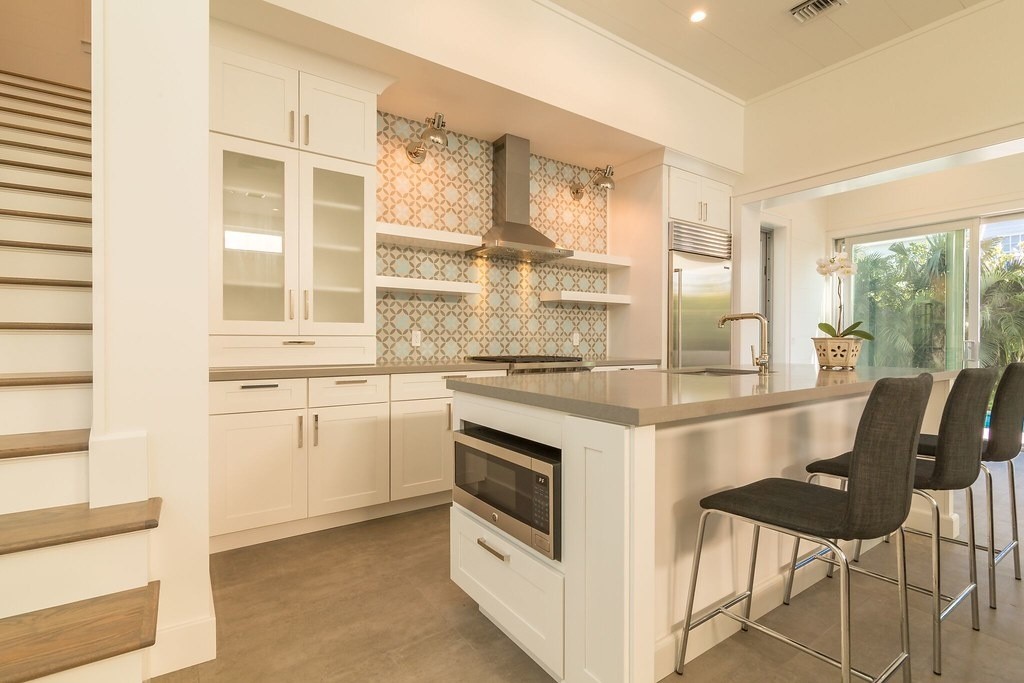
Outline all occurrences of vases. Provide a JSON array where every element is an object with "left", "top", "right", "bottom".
[{"left": 811, "top": 337, "right": 863, "bottom": 369}]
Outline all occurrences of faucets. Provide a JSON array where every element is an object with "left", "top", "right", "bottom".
[{"left": 718, "top": 313, "right": 768, "bottom": 376}]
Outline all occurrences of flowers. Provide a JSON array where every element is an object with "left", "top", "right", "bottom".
[{"left": 815, "top": 251, "right": 875, "bottom": 341}]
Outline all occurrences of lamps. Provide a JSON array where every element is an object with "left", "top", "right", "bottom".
[
  {"left": 570, "top": 165, "right": 616, "bottom": 200},
  {"left": 406, "top": 112, "right": 448, "bottom": 164}
]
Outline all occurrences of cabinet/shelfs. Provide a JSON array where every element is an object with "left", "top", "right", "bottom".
[
  {"left": 540, "top": 251, "right": 632, "bottom": 304},
  {"left": 207, "top": 366, "right": 391, "bottom": 554},
  {"left": 210, "top": 16, "right": 397, "bottom": 165},
  {"left": 387, "top": 363, "right": 510, "bottom": 515},
  {"left": 667, "top": 165, "right": 731, "bottom": 233},
  {"left": 209, "top": 130, "right": 378, "bottom": 367},
  {"left": 376, "top": 221, "right": 483, "bottom": 294}
]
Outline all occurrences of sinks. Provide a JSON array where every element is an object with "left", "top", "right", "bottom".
[{"left": 647, "top": 368, "right": 779, "bottom": 376}]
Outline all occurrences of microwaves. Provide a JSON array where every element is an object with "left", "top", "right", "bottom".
[{"left": 451, "top": 426, "right": 561, "bottom": 563}]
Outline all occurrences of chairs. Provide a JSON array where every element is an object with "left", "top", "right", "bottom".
[{"left": 675, "top": 361, "right": 1024, "bottom": 683}]
